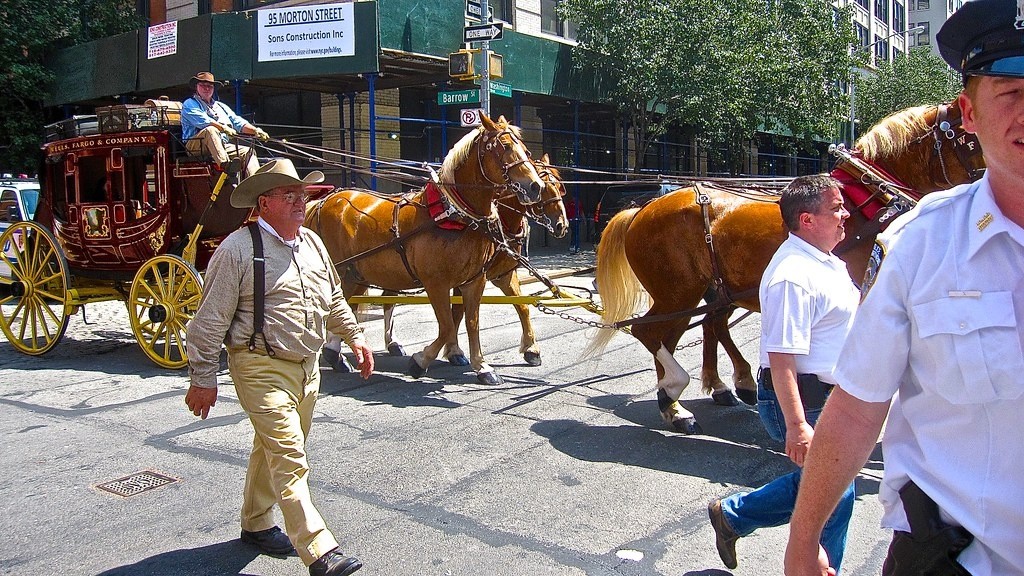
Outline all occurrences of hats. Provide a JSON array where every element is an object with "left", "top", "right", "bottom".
[
  {"left": 936, "top": 1, "right": 1024, "bottom": 88},
  {"left": 230, "top": 159, "right": 325, "bottom": 208},
  {"left": 189, "top": 72, "right": 224, "bottom": 91}
]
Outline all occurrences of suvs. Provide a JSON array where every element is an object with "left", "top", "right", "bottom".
[{"left": 595, "top": 183, "right": 682, "bottom": 245}]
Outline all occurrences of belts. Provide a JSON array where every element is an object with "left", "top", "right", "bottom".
[{"left": 760, "top": 367, "right": 832, "bottom": 393}]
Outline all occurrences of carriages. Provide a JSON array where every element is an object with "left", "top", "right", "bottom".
[{"left": 1, "top": 95, "right": 984, "bottom": 439}]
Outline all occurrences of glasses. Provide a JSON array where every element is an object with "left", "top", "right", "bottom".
[
  {"left": 198, "top": 84, "right": 213, "bottom": 89},
  {"left": 269, "top": 193, "right": 310, "bottom": 204}
]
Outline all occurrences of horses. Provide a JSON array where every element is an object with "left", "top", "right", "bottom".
[
  {"left": 580, "top": 97, "right": 987, "bottom": 436},
  {"left": 301, "top": 109, "right": 545, "bottom": 385},
  {"left": 381, "top": 153, "right": 568, "bottom": 367}
]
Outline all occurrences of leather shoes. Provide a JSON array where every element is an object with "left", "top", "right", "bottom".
[
  {"left": 310, "top": 551, "right": 362, "bottom": 576},
  {"left": 241, "top": 526, "right": 296, "bottom": 557}
]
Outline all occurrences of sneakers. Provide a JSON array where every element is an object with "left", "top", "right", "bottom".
[{"left": 709, "top": 499, "right": 737, "bottom": 569}]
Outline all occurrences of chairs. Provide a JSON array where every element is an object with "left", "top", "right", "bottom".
[{"left": 168, "top": 122, "right": 213, "bottom": 163}]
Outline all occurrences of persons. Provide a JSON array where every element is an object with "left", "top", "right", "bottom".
[
  {"left": 565, "top": 194, "right": 586, "bottom": 252},
  {"left": 782, "top": 0, "right": 1024, "bottom": 576},
  {"left": 185, "top": 157, "right": 374, "bottom": 576},
  {"left": 709, "top": 175, "right": 862, "bottom": 576},
  {"left": 181, "top": 72, "right": 270, "bottom": 179}
]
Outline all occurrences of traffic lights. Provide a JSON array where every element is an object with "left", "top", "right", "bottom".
[{"left": 448, "top": 50, "right": 474, "bottom": 79}]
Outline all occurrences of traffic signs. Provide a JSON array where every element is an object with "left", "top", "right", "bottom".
[
  {"left": 463, "top": 22, "right": 504, "bottom": 44},
  {"left": 465, "top": 0, "right": 494, "bottom": 23}
]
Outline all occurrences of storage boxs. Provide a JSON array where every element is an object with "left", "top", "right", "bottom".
[{"left": 95, "top": 105, "right": 155, "bottom": 132}]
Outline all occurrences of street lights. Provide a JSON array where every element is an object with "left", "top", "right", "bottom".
[{"left": 845, "top": 26, "right": 924, "bottom": 152}]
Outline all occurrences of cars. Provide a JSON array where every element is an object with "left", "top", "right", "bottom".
[{"left": 0, "top": 174, "right": 41, "bottom": 278}]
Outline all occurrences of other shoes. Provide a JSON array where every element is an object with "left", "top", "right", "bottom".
[
  {"left": 576, "top": 247, "right": 582, "bottom": 255},
  {"left": 569, "top": 246, "right": 575, "bottom": 254},
  {"left": 221, "top": 157, "right": 242, "bottom": 176}
]
